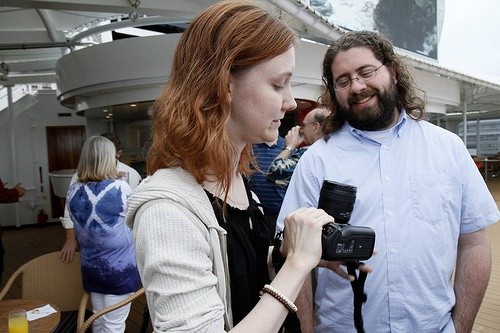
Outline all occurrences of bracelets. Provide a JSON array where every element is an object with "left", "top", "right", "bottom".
[
  {"left": 285, "top": 146, "right": 293, "bottom": 152},
  {"left": 262, "top": 284, "right": 299, "bottom": 313}
]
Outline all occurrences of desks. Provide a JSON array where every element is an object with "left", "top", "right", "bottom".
[
  {"left": 474, "top": 159, "right": 500, "bottom": 182},
  {"left": 0, "top": 298, "right": 60, "bottom": 333}
]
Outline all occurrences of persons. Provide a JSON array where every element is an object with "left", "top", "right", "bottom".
[
  {"left": 59, "top": 131, "right": 142, "bottom": 264},
  {"left": 266, "top": 107, "right": 333, "bottom": 190},
  {"left": 0, "top": 176, "right": 26, "bottom": 288},
  {"left": 273, "top": 29, "right": 500, "bottom": 333},
  {"left": 125, "top": 0, "right": 377, "bottom": 333},
  {"left": 66, "top": 136, "right": 143, "bottom": 333},
  {"left": 246, "top": 136, "right": 297, "bottom": 236}
]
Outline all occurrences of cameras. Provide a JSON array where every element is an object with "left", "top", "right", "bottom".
[{"left": 314, "top": 179, "right": 376, "bottom": 261}]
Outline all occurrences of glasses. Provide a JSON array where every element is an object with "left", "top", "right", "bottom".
[
  {"left": 299, "top": 120, "right": 322, "bottom": 129},
  {"left": 331, "top": 61, "right": 383, "bottom": 92}
]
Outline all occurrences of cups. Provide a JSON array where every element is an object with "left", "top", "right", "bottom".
[
  {"left": 284, "top": 128, "right": 305, "bottom": 146},
  {"left": 8, "top": 308, "right": 28, "bottom": 333}
]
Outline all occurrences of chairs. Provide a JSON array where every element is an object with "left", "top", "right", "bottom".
[
  {"left": 0, "top": 251, "right": 90, "bottom": 333},
  {"left": 79, "top": 288, "right": 145, "bottom": 333}
]
